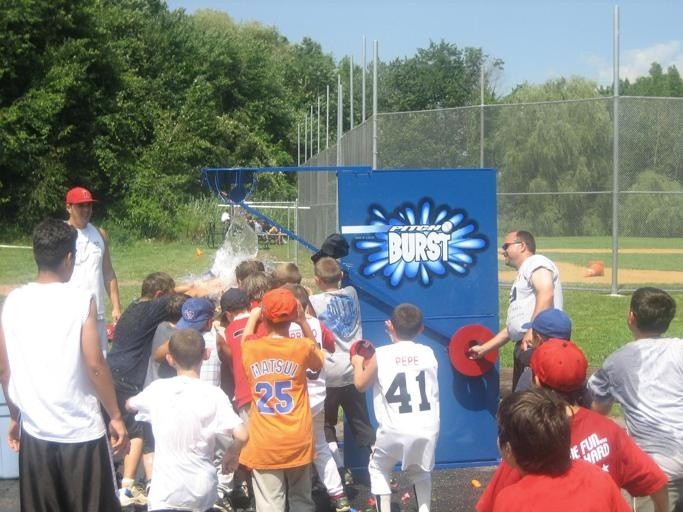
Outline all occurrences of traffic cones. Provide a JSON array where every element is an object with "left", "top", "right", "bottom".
[
  {"left": 194, "top": 247, "right": 203, "bottom": 256},
  {"left": 582, "top": 259, "right": 605, "bottom": 278}
]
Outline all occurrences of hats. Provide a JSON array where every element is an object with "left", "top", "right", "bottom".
[
  {"left": 176, "top": 298, "right": 215, "bottom": 332},
  {"left": 260, "top": 287, "right": 299, "bottom": 324},
  {"left": 66, "top": 187, "right": 97, "bottom": 205},
  {"left": 522, "top": 308, "right": 571, "bottom": 340},
  {"left": 310, "top": 234, "right": 349, "bottom": 263},
  {"left": 349, "top": 340, "right": 375, "bottom": 368},
  {"left": 212, "top": 288, "right": 250, "bottom": 322},
  {"left": 528, "top": 338, "right": 587, "bottom": 392}
]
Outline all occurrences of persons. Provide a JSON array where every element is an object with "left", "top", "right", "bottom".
[
  {"left": 1, "top": 217, "right": 131, "bottom": 511},
  {"left": 468, "top": 230, "right": 563, "bottom": 389},
  {"left": 221, "top": 210, "right": 231, "bottom": 223},
  {"left": 101, "top": 257, "right": 374, "bottom": 512},
  {"left": 489, "top": 388, "right": 628, "bottom": 512},
  {"left": 513, "top": 306, "right": 591, "bottom": 408},
  {"left": 239, "top": 208, "right": 280, "bottom": 242},
  {"left": 476, "top": 339, "right": 672, "bottom": 512},
  {"left": 590, "top": 287, "right": 682, "bottom": 512},
  {"left": 64, "top": 188, "right": 122, "bottom": 359},
  {"left": 349, "top": 303, "right": 439, "bottom": 512}
]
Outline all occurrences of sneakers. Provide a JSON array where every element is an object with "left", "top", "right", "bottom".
[
  {"left": 211, "top": 481, "right": 250, "bottom": 512},
  {"left": 328, "top": 483, "right": 360, "bottom": 512},
  {"left": 118, "top": 485, "right": 148, "bottom": 507}
]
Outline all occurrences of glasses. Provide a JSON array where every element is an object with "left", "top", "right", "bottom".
[{"left": 503, "top": 241, "right": 531, "bottom": 250}]
